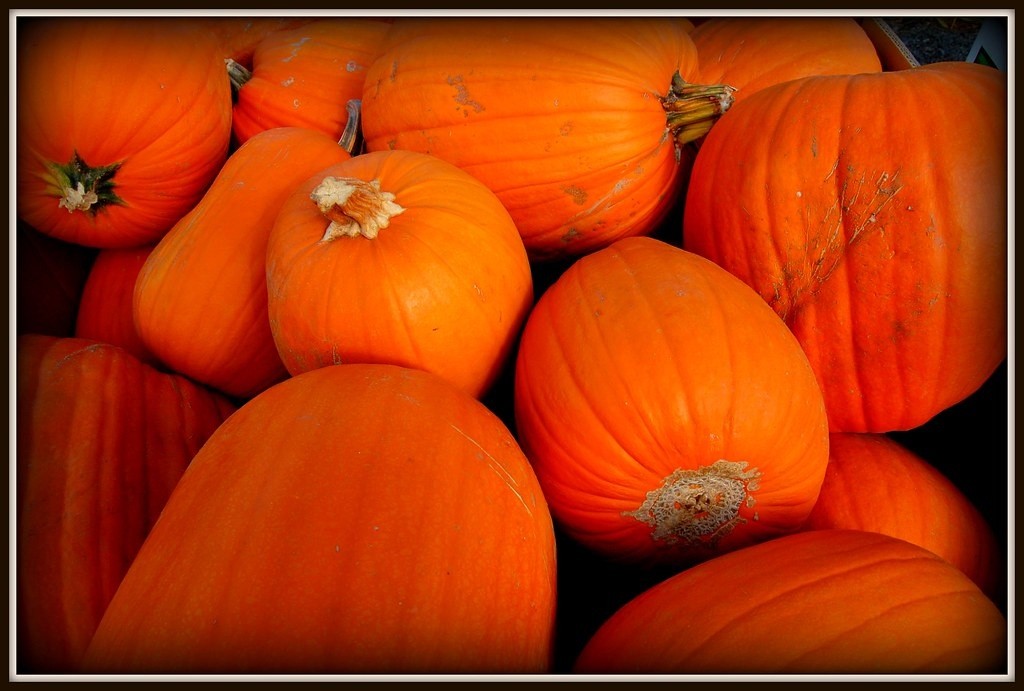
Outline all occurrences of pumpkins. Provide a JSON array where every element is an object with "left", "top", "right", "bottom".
[{"left": 17, "top": 17, "right": 1006, "bottom": 675}]
